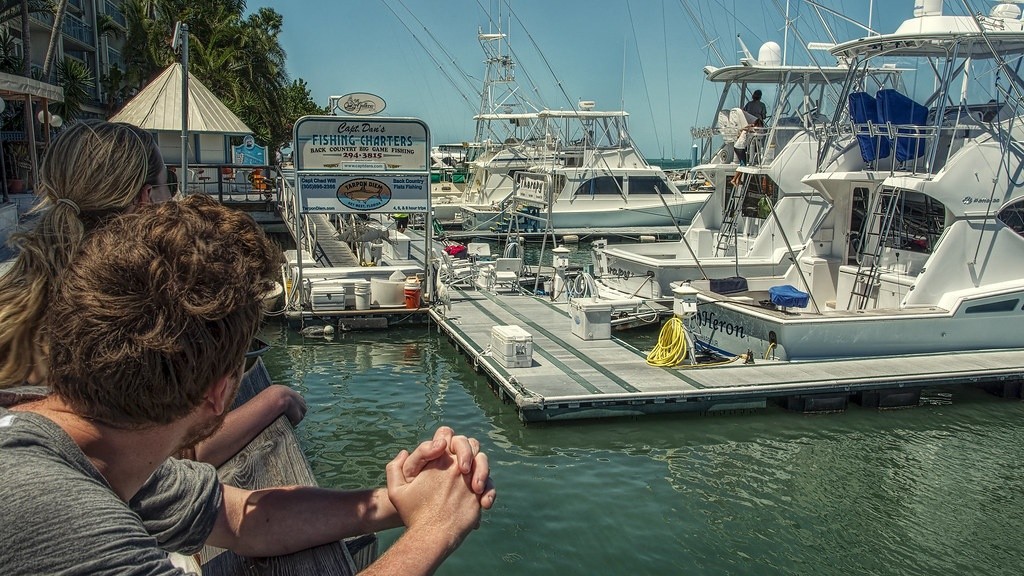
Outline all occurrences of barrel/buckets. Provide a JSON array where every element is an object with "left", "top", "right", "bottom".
[
  {"left": 404, "top": 276, "right": 421, "bottom": 308},
  {"left": 354, "top": 281, "right": 371, "bottom": 310}
]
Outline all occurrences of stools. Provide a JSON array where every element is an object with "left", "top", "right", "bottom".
[
  {"left": 690, "top": 228, "right": 719, "bottom": 258},
  {"left": 221, "top": 167, "right": 237, "bottom": 202},
  {"left": 799, "top": 256, "right": 840, "bottom": 310},
  {"left": 254, "top": 177, "right": 266, "bottom": 202},
  {"left": 769, "top": 285, "right": 811, "bottom": 313},
  {"left": 198, "top": 177, "right": 213, "bottom": 199}
]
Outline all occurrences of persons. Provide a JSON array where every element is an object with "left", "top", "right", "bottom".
[
  {"left": 0, "top": 199, "right": 496, "bottom": 576},
  {"left": 742, "top": 89, "right": 766, "bottom": 126},
  {"left": 394, "top": 214, "right": 409, "bottom": 232},
  {"left": 0, "top": 122, "right": 308, "bottom": 466},
  {"left": 730, "top": 122, "right": 760, "bottom": 186},
  {"left": 272, "top": 148, "right": 283, "bottom": 166}
]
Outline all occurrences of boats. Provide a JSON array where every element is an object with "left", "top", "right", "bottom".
[
  {"left": 668, "top": 0, "right": 1023, "bottom": 367},
  {"left": 571, "top": 0, "right": 897, "bottom": 329},
  {"left": 458, "top": 101, "right": 713, "bottom": 230}
]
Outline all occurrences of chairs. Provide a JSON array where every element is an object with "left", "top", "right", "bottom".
[{"left": 431, "top": 242, "right": 523, "bottom": 298}]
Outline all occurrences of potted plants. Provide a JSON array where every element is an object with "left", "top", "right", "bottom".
[{"left": 4, "top": 142, "right": 28, "bottom": 194}]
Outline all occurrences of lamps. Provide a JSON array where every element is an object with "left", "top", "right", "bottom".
[
  {"left": 50, "top": 116, "right": 62, "bottom": 133},
  {"left": 38, "top": 110, "right": 53, "bottom": 126}
]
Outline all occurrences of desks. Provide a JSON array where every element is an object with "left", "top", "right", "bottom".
[
  {"left": 236, "top": 168, "right": 253, "bottom": 203},
  {"left": 188, "top": 167, "right": 204, "bottom": 198}
]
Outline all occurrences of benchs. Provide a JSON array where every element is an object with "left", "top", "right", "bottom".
[
  {"left": 712, "top": 232, "right": 757, "bottom": 258},
  {"left": 840, "top": 246, "right": 930, "bottom": 288}
]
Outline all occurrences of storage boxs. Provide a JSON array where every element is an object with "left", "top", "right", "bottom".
[
  {"left": 310, "top": 287, "right": 347, "bottom": 311},
  {"left": 382, "top": 229, "right": 412, "bottom": 260},
  {"left": 491, "top": 324, "right": 533, "bottom": 368},
  {"left": 571, "top": 298, "right": 612, "bottom": 340}
]
